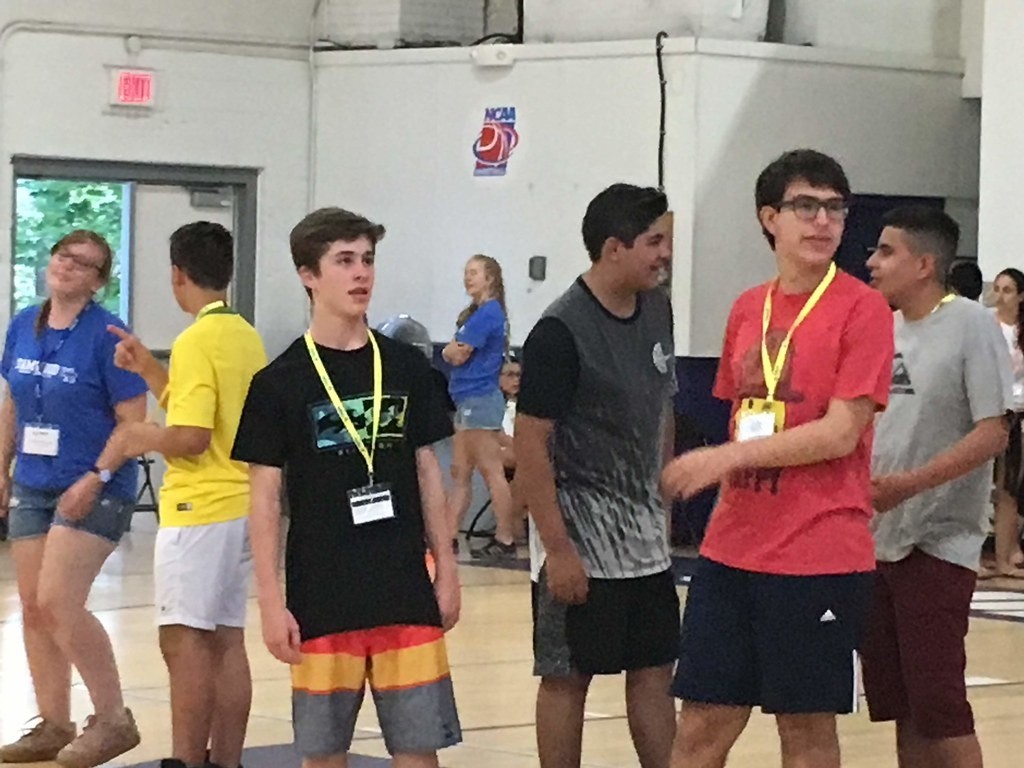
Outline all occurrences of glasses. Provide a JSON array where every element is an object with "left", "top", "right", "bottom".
[
  {"left": 55, "top": 246, "right": 104, "bottom": 275},
  {"left": 771, "top": 195, "right": 851, "bottom": 222}
]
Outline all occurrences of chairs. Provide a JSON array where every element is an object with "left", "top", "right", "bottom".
[{"left": 129, "top": 455, "right": 162, "bottom": 531}]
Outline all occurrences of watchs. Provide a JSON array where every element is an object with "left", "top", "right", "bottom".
[{"left": 88, "top": 464, "right": 112, "bottom": 484}]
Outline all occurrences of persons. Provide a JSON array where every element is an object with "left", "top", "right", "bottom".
[
  {"left": 853, "top": 201, "right": 1024, "bottom": 767},
  {"left": 510, "top": 180, "right": 679, "bottom": 767},
  {"left": 105, "top": 219, "right": 268, "bottom": 768},
  {"left": 427, "top": 252, "right": 532, "bottom": 562},
  {"left": 665, "top": 147, "right": 895, "bottom": 767},
  {"left": 0, "top": 228, "right": 152, "bottom": 768},
  {"left": 227, "top": 204, "right": 465, "bottom": 768}
]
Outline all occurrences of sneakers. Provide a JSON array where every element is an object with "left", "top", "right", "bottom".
[
  {"left": 56, "top": 707, "right": 141, "bottom": 768},
  {"left": 470, "top": 539, "right": 518, "bottom": 560},
  {"left": 0, "top": 715, "right": 77, "bottom": 762}
]
